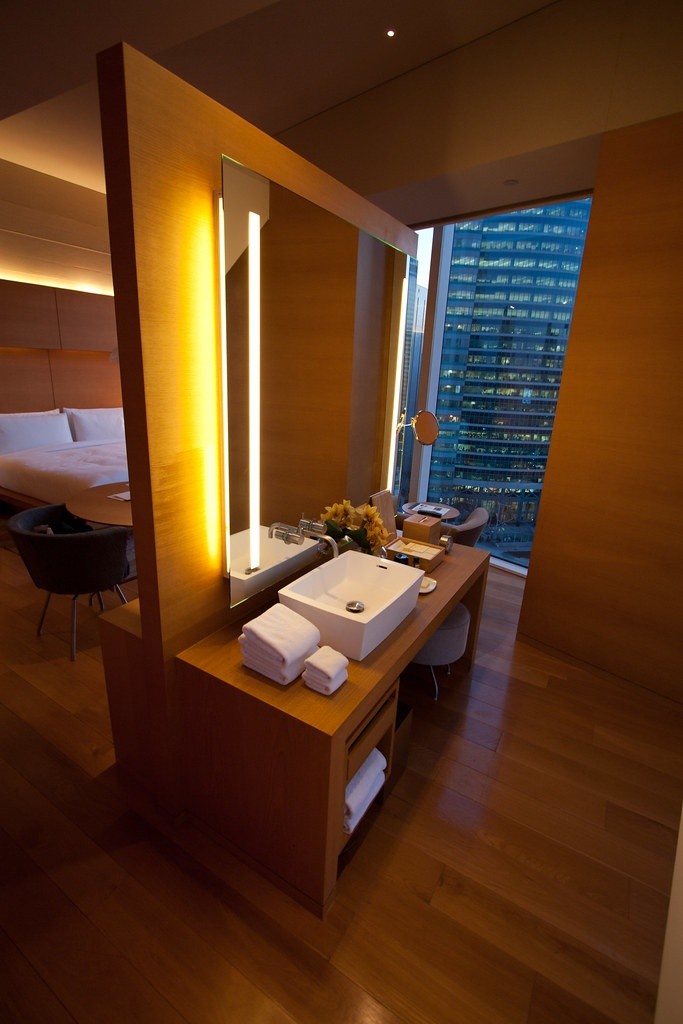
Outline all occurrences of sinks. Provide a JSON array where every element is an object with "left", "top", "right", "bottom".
[
  {"left": 229, "top": 524, "right": 320, "bottom": 608},
  {"left": 278, "top": 550, "right": 426, "bottom": 662}
]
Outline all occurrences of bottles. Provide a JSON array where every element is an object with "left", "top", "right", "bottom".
[
  {"left": 394, "top": 553, "right": 409, "bottom": 565},
  {"left": 412, "top": 557, "right": 420, "bottom": 569}
]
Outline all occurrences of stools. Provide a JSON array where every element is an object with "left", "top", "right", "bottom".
[{"left": 411, "top": 601, "right": 471, "bottom": 701}]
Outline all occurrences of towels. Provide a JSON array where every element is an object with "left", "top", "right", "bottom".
[
  {"left": 237, "top": 602, "right": 321, "bottom": 686},
  {"left": 343, "top": 747, "right": 387, "bottom": 835},
  {"left": 302, "top": 645, "right": 350, "bottom": 697}
]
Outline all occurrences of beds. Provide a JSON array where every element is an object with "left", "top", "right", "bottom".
[{"left": 0, "top": 346, "right": 128, "bottom": 555}]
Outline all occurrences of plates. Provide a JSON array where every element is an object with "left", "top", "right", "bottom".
[{"left": 419, "top": 576, "right": 437, "bottom": 594}]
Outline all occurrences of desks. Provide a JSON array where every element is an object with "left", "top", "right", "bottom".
[
  {"left": 66, "top": 481, "right": 133, "bottom": 604},
  {"left": 401, "top": 501, "right": 460, "bottom": 520}
]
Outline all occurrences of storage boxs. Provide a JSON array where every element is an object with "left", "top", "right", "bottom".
[{"left": 385, "top": 536, "right": 446, "bottom": 574}]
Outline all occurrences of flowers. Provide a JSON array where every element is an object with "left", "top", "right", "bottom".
[
  {"left": 317, "top": 499, "right": 353, "bottom": 545},
  {"left": 345, "top": 505, "right": 390, "bottom": 557}
]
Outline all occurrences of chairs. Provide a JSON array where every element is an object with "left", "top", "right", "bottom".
[
  {"left": 391, "top": 496, "right": 410, "bottom": 530},
  {"left": 6, "top": 502, "right": 130, "bottom": 662},
  {"left": 441, "top": 506, "right": 489, "bottom": 547}
]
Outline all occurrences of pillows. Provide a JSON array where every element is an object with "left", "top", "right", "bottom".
[
  {"left": 63, "top": 408, "right": 123, "bottom": 441},
  {"left": 0, "top": 412, "right": 74, "bottom": 454},
  {"left": 72, "top": 411, "right": 125, "bottom": 442}
]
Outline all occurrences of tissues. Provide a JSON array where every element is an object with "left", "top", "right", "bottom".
[{"left": 402, "top": 513, "right": 442, "bottom": 546}]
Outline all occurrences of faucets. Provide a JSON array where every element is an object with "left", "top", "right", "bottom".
[
  {"left": 268, "top": 522, "right": 299, "bottom": 539},
  {"left": 298, "top": 528, "right": 339, "bottom": 558}
]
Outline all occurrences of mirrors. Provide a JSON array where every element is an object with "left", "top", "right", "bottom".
[
  {"left": 395, "top": 407, "right": 439, "bottom": 445},
  {"left": 212, "top": 151, "right": 419, "bottom": 610}
]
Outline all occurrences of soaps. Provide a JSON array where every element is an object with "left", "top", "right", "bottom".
[{"left": 420, "top": 579, "right": 429, "bottom": 588}]
